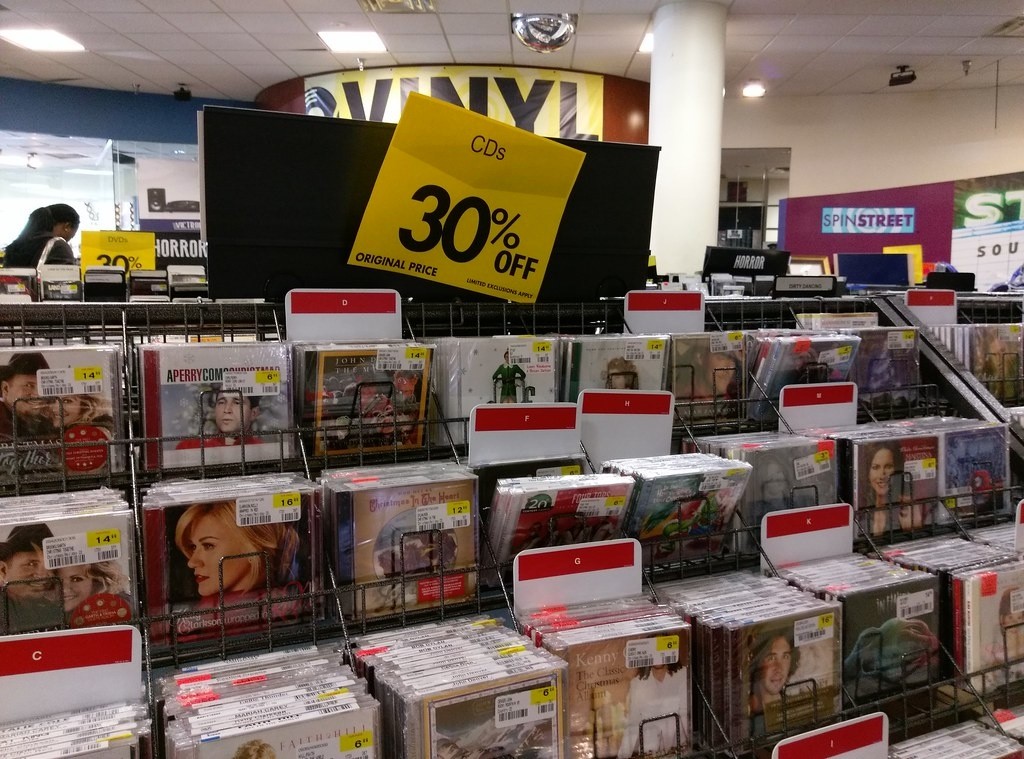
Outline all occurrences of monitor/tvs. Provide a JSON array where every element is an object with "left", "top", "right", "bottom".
[
  {"left": 702, "top": 246, "right": 836, "bottom": 301},
  {"left": 926, "top": 272, "right": 975, "bottom": 292}
]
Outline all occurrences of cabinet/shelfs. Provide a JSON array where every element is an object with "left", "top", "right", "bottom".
[{"left": 0, "top": 256, "right": 1024, "bottom": 759}]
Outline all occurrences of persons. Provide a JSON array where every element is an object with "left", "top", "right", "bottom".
[
  {"left": 3, "top": 203, "right": 81, "bottom": 267},
  {"left": 2, "top": 339, "right": 1022, "bottom": 759}
]
